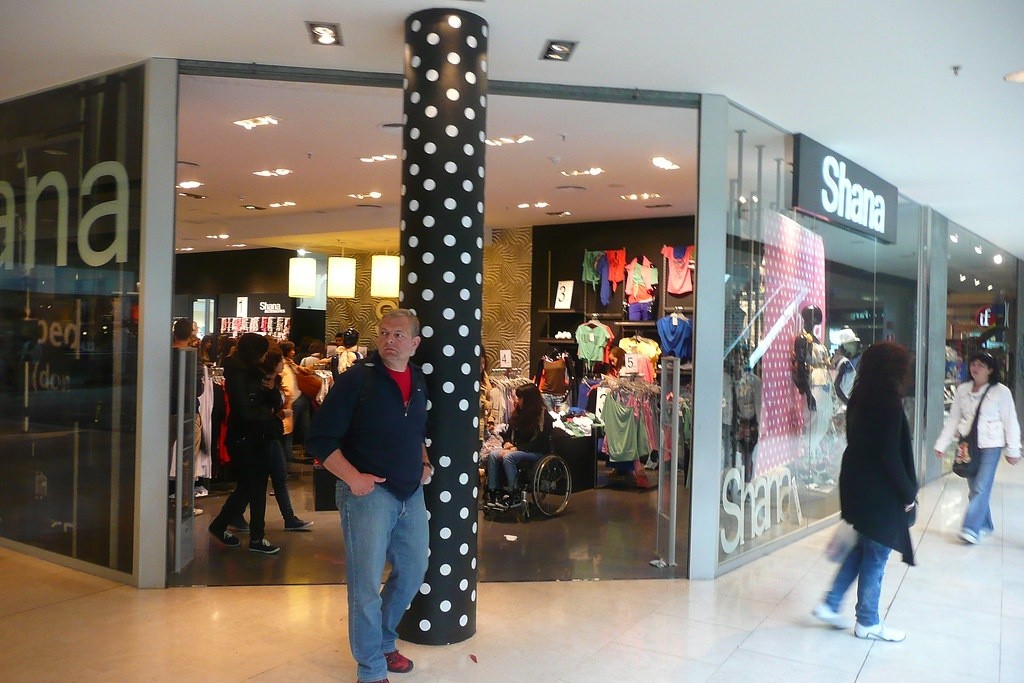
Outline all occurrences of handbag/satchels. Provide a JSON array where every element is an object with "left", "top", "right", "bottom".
[
  {"left": 953, "top": 431, "right": 980, "bottom": 478},
  {"left": 824, "top": 520, "right": 859, "bottom": 564},
  {"left": 285, "top": 357, "right": 322, "bottom": 399}
]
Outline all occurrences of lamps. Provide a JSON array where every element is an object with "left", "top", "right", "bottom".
[
  {"left": 326, "top": 247, "right": 356, "bottom": 298},
  {"left": 370, "top": 244, "right": 400, "bottom": 297},
  {"left": 288, "top": 251, "right": 316, "bottom": 297}
]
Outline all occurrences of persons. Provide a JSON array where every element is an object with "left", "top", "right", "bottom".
[
  {"left": 812, "top": 341, "right": 918, "bottom": 642},
  {"left": 278, "top": 340, "right": 301, "bottom": 459},
  {"left": 293, "top": 337, "right": 324, "bottom": 367},
  {"left": 721, "top": 346, "right": 762, "bottom": 502},
  {"left": 791, "top": 305, "right": 834, "bottom": 490},
  {"left": 208, "top": 333, "right": 281, "bottom": 553},
  {"left": 829, "top": 328, "right": 860, "bottom": 420},
  {"left": 305, "top": 308, "right": 430, "bottom": 683},
  {"left": 486, "top": 383, "right": 552, "bottom": 509},
  {"left": 198, "top": 335, "right": 212, "bottom": 362},
  {"left": 169, "top": 321, "right": 204, "bottom": 516},
  {"left": 335, "top": 332, "right": 343, "bottom": 347},
  {"left": 934, "top": 352, "right": 1022, "bottom": 544},
  {"left": 227, "top": 351, "right": 314, "bottom": 530}
]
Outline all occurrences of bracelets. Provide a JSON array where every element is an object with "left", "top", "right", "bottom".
[{"left": 423, "top": 462, "right": 431, "bottom": 468}]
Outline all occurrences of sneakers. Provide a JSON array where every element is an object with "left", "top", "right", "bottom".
[
  {"left": 208, "top": 527, "right": 240, "bottom": 546},
  {"left": 227, "top": 516, "right": 250, "bottom": 532},
  {"left": 487, "top": 492, "right": 496, "bottom": 507},
  {"left": 195, "top": 484, "right": 208, "bottom": 497},
  {"left": 357, "top": 678, "right": 389, "bottom": 683},
  {"left": 812, "top": 602, "right": 853, "bottom": 629},
  {"left": 496, "top": 495, "right": 522, "bottom": 509},
  {"left": 854, "top": 619, "right": 906, "bottom": 642},
  {"left": 248, "top": 538, "right": 280, "bottom": 554},
  {"left": 285, "top": 516, "right": 313, "bottom": 530},
  {"left": 384, "top": 650, "right": 413, "bottom": 673}
]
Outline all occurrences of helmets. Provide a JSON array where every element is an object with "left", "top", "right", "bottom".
[{"left": 342, "top": 328, "right": 360, "bottom": 348}]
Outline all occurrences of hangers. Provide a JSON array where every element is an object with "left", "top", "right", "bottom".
[
  {"left": 487, "top": 368, "right": 533, "bottom": 390},
  {"left": 201, "top": 366, "right": 226, "bottom": 385},
  {"left": 629, "top": 330, "right": 645, "bottom": 343},
  {"left": 580, "top": 313, "right": 603, "bottom": 327},
  {"left": 670, "top": 306, "right": 690, "bottom": 325},
  {"left": 605, "top": 371, "right": 684, "bottom": 407}
]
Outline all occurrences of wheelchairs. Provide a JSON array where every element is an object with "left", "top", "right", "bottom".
[{"left": 480, "top": 430, "right": 573, "bottom": 524}]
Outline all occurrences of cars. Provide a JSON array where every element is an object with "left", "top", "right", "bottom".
[{"left": 0, "top": 304, "right": 136, "bottom": 436}]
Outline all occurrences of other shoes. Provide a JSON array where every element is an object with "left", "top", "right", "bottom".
[
  {"left": 193, "top": 507, "right": 203, "bottom": 516},
  {"left": 956, "top": 529, "right": 994, "bottom": 544}
]
